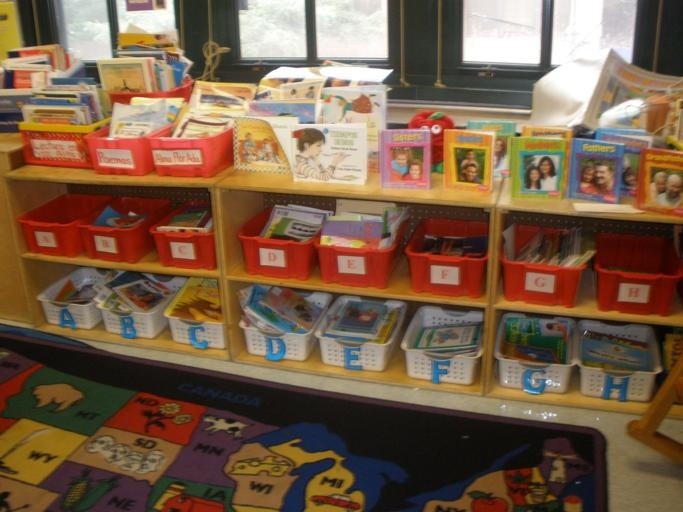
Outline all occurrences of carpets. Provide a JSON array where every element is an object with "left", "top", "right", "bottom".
[{"left": 1, "top": 333, "right": 610, "bottom": 511}]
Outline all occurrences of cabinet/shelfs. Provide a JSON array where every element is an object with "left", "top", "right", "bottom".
[
  {"left": 482, "top": 167, "right": 682, "bottom": 416},
  {"left": 214, "top": 162, "right": 500, "bottom": 398},
  {"left": 0, "top": 157, "right": 234, "bottom": 364}
]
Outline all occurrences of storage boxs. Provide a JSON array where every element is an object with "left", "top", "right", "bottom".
[
  {"left": 309, "top": 214, "right": 405, "bottom": 291},
  {"left": 107, "top": 77, "right": 193, "bottom": 108}
]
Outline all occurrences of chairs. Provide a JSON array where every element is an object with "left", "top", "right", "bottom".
[{"left": 626, "top": 355, "right": 682, "bottom": 464}]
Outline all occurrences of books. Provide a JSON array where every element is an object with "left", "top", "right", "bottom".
[{"left": 1, "top": 21, "right": 682, "bottom": 376}]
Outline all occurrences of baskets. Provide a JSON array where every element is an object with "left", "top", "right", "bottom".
[
  {"left": 314, "top": 231, "right": 402, "bottom": 289},
  {"left": 18, "top": 112, "right": 113, "bottom": 169},
  {"left": 237, "top": 209, "right": 313, "bottom": 280},
  {"left": 96, "top": 272, "right": 187, "bottom": 338},
  {"left": 238, "top": 286, "right": 332, "bottom": 363},
  {"left": 401, "top": 305, "right": 481, "bottom": 389},
  {"left": 36, "top": 267, "right": 121, "bottom": 330},
  {"left": 574, "top": 320, "right": 663, "bottom": 403},
  {"left": 315, "top": 294, "right": 406, "bottom": 373},
  {"left": 493, "top": 311, "right": 576, "bottom": 396},
  {"left": 164, "top": 277, "right": 224, "bottom": 351}
]
